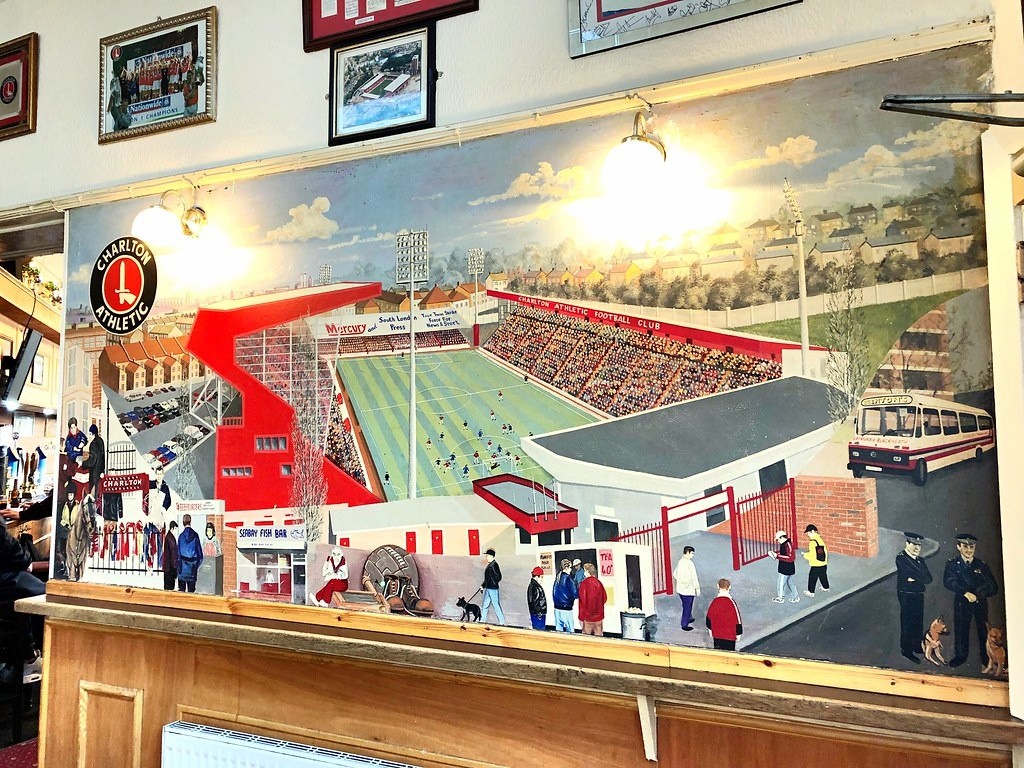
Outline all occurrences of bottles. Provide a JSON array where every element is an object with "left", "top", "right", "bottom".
[{"left": 11, "top": 479, "right": 19, "bottom": 508}]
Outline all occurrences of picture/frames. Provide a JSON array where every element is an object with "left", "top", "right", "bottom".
[
  {"left": 98, "top": 5, "right": 218, "bottom": 146},
  {"left": 301, "top": 0, "right": 479, "bottom": 53},
  {"left": 567, "top": 0, "right": 804, "bottom": 60},
  {"left": 0, "top": 32, "right": 39, "bottom": 142},
  {"left": 328, "top": 21, "right": 435, "bottom": 147}
]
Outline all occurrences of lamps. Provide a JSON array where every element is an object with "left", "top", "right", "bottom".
[
  {"left": 612, "top": 112, "right": 666, "bottom": 176},
  {"left": 131, "top": 192, "right": 207, "bottom": 241}
]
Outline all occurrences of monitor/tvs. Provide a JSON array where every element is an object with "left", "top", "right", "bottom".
[{"left": 3, "top": 328, "right": 43, "bottom": 402}]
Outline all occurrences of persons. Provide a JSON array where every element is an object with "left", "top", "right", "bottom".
[{"left": 0, "top": 487, "right": 53, "bottom": 683}]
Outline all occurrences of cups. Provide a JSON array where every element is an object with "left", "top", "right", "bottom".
[{"left": 0, "top": 495, "right": 7, "bottom": 510}]
